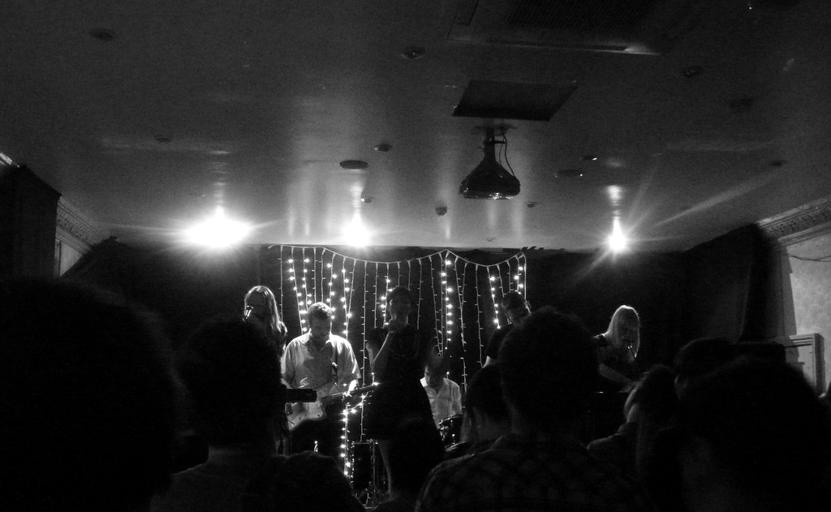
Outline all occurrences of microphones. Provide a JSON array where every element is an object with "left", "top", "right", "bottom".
[{"left": 389, "top": 311, "right": 401, "bottom": 335}]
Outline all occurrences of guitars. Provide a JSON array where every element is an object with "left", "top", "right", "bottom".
[{"left": 285, "top": 381, "right": 382, "bottom": 432}]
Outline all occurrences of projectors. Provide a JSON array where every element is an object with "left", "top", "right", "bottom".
[{"left": 460, "top": 176, "right": 520, "bottom": 201}]
